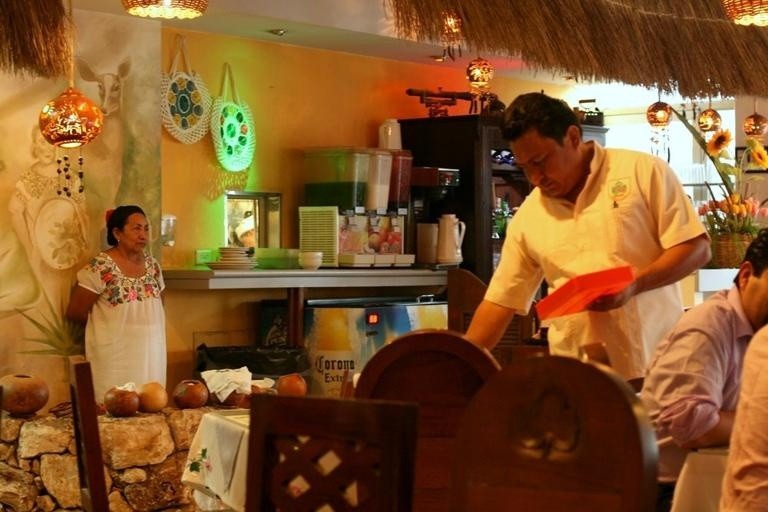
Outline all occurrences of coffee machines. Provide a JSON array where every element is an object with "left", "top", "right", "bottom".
[{"left": 411, "top": 165, "right": 466, "bottom": 269}]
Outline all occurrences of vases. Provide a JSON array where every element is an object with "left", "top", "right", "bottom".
[{"left": 713, "top": 232, "right": 753, "bottom": 268}]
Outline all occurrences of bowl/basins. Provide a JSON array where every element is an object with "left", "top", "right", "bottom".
[{"left": 299, "top": 251, "right": 325, "bottom": 270}]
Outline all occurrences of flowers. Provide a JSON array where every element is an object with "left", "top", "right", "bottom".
[{"left": 668, "top": 107, "right": 768, "bottom": 263}]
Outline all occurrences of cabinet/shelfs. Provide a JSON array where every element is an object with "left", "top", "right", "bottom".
[{"left": 396, "top": 114, "right": 608, "bottom": 287}]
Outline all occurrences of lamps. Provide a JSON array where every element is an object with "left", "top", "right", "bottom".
[
  {"left": 431, "top": 8, "right": 464, "bottom": 62},
  {"left": 698, "top": 92, "right": 721, "bottom": 132},
  {"left": 722, "top": 0, "right": 768, "bottom": 28},
  {"left": 466, "top": 47, "right": 496, "bottom": 115},
  {"left": 646, "top": 82, "right": 671, "bottom": 128},
  {"left": 39, "top": 0, "right": 104, "bottom": 200},
  {"left": 744, "top": 95, "right": 767, "bottom": 141},
  {"left": 121, "top": 0, "right": 208, "bottom": 20}
]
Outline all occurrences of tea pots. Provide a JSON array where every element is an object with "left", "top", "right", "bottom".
[{"left": 437, "top": 210, "right": 465, "bottom": 264}]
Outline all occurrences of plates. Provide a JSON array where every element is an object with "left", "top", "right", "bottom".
[{"left": 206, "top": 246, "right": 299, "bottom": 272}]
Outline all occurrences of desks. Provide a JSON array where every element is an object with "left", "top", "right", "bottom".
[
  {"left": 161, "top": 268, "right": 445, "bottom": 350},
  {"left": 692, "top": 268, "right": 741, "bottom": 309}
]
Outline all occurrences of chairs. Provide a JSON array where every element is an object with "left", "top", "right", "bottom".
[
  {"left": 63, "top": 353, "right": 111, "bottom": 512},
  {"left": 458, "top": 355, "right": 660, "bottom": 512},
  {"left": 445, "top": 269, "right": 534, "bottom": 359},
  {"left": 668, "top": 447, "right": 730, "bottom": 512},
  {"left": 245, "top": 394, "right": 417, "bottom": 512},
  {"left": 351, "top": 328, "right": 503, "bottom": 510}
]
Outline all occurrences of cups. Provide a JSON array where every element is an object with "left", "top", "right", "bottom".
[
  {"left": 416, "top": 223, "right": 438, "bottom": 264},
  {"left": 378, "top": 118, "right": 402, "bottom": 150}
]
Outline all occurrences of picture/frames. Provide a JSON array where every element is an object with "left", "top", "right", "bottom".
[{"left": 735, "top": 147, "right": 768, "bottom": 173}]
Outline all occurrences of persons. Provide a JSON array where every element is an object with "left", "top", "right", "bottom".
[
  {"left": 714, "top": 320, "right": 768, "bottom": 511},
  {"left": 63, "top": 203, "right": 166, "bottom": 406},
  {"left": 638, "top": 223, "right": 768, "bottom": 512},
  {"left": 459, "top": 92, "right": 713, "bottom": 395}
]
